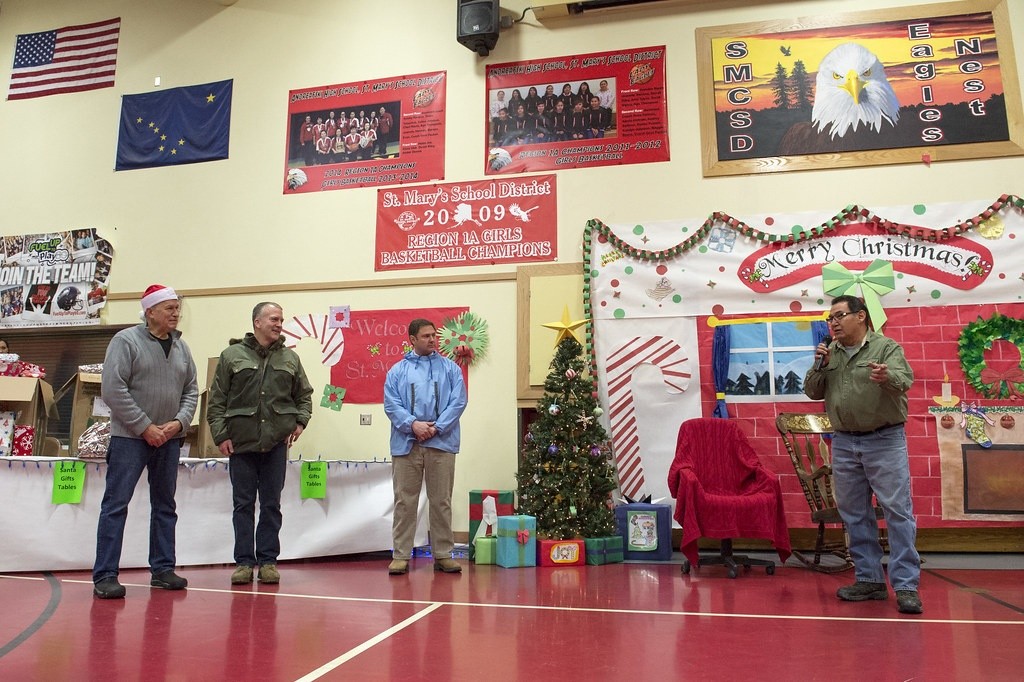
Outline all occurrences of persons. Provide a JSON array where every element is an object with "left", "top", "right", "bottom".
[
  {"left": 93, "top": 286, "right": 199, "bottom": 599},
  {"left": 0, "top": 288, "right": 23, "bottom": 319},
  {"left": 489, "top": 77, "right": 615, "bottom": 146},
  {"left": 88, "top": 281, "right": 107, "bottom": 304},
  {"left": 0, "top": 338, "right": 9, "bottom": 354},
  {"left": 299, "top": 105, "right": 394, "bottom": 166},
  {"left": 802, "top": 294, "right": 925, "bottom": 615},
  {"left": 382, "top": 319, "right": 468, "bottom": 575},
  {"left": 207, "top": 301, "right": 314, "bottom": 583},
  {"left": 75, "top": 230, "right": 94, "bottom": 249},
  {"left": 95, "top": 252, "right": 112, "bottom": 283}
]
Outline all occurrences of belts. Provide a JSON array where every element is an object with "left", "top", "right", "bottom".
[{"left": 839, "top": 422, "right": 902, "bottom": 437}]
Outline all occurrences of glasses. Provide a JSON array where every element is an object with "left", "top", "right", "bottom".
[{"left": 825, "top": 311, "right": 859, "bottom": 324}]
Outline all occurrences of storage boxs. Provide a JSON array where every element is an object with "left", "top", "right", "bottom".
[
  {"left": 468, "top": 489, "right": 514, "bottom": 561},
  {"left": 496, "top": 514, "right": 537, "bottom": 568},
  {"left": 60, "top": 445, "right": 70, "bottom": 458},
  {"left": 52, "top": 371, "right": 111, "bottom": 457},
  {"left": 475, "top": 537, "right": 497, "bottom": 565},
  {"left": 0, "top": 374, "right": 54, "bottom": 455},
  {"left": 584, "top": 536, "right": 623, "bottom": 565},
  {"left": 195, "top": 357, "right": 232, "bottom": 459},
  {"left": 11, "top": 425, "right": 36, "bottom": 456},
  {"left": 179, "top": 424, "right": 199, "bottom": 458},
  {"left": 537, "top": 539, "right": 585, "bottom": 567},
  {"left": 0, "top": 411, "right": 17, "bottom": 456},
  {"left": 616, "top": 502, "right": 672, "bottom": 561}
]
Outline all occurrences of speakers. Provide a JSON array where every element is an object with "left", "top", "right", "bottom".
[{"left": 457, "top": 0, "right": 500, "bottom": 51}]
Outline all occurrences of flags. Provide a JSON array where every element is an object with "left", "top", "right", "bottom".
[
  {"left": 115, "top": 78, "right": 233, "bottom": 171},
  {"left": 7, "top": 16, "right": 121, "bottom": 101}
]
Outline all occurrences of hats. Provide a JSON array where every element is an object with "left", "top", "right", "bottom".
[{"left": 141, "top": 284, "right": 177, "bottom": 321}]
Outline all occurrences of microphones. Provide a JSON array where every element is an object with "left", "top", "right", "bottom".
[{"left": 813, "top": 334, "right": 832, "bottom": 373}]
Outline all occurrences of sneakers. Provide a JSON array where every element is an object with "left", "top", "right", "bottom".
[
  {"left": 389, "top": 559, "right": 409, "bottom": 574},
  {"left": 896, "top": 590, "right": 924, "bottom": 613},
  {"left": 93, "top": 577, "right": 126, "bottom": 599},
  {"left": 231, "top": 565, "right": 253, "bottom": 583},
  {"left": 151, "top": 570, "right": 188, "bottom": 589},
  {"left": 434, "top": 558, "right": 462, "bottom": 571},
  {"left": 257, "top": 564, "right": 280, "bottom": 582},
  {"left": 837, "top": 581, "right": 888, "bottom": 601}
]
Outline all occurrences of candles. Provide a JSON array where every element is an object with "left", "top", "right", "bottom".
[{"left": 941, "top": 373, "right": 951, "bottom": 402}]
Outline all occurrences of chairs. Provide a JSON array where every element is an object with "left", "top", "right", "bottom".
[
  {"left": 775, "top": 412, "right": 926, "bottom": 573},
  {"left": 42, "top": 437, "right": 60, "bottom": 457},
  {"left": 667, "top": 417, "right": 793, "bottom": 578}
]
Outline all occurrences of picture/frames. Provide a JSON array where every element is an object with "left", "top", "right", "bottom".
[{"left": 695, "top": 0, "right": 1024, "bottom": 177}]
